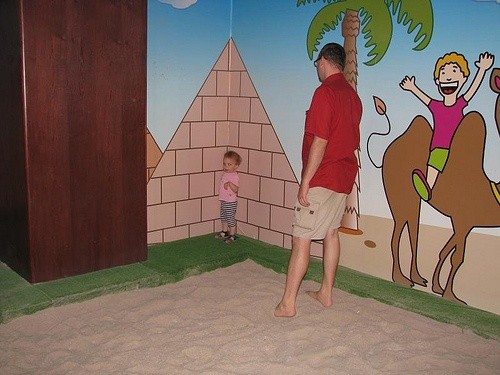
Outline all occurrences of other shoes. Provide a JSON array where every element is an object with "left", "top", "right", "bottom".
[
  {"left": 224, "top": 236, "right": 236, "bottom": 243},
  {"left": 214, "top": 231, "right": 228, "bottom": 238}
]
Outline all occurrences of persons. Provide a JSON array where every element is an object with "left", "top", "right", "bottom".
[
  {"left": 274, "top": 42, "right": 363, "bottom": 318},
  {"left": 214, "top": 151, "right": 242, "bottom": 244}
]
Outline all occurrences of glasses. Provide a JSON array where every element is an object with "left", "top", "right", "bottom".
[{"left": 314, "top": 56, "right": 327, "bottom": 67}]
